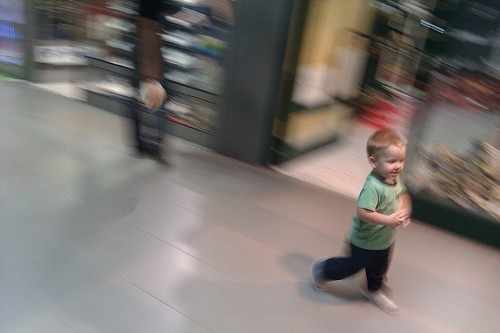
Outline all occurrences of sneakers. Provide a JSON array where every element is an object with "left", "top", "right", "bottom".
[
  {"left": 311, "top": 260, "right": 330, "bottom": 291},
  {"left": 361, "top": 288, "right": 396, "bottom": 311}
]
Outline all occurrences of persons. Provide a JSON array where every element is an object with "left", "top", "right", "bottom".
[
  {"left": 311, "top": 129, "right": 412, "bottom": 313},
  {"left": 126, "top": 1, "right": 184, "bottom": 171}
]
